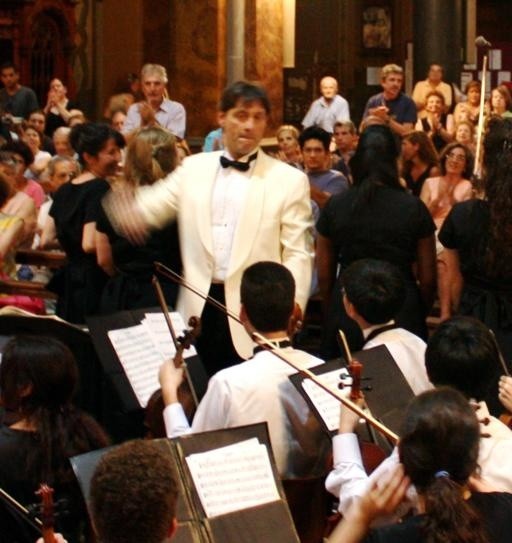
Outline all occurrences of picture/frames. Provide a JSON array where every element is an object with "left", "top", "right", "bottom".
[{"left": 359, "top": 0, "right": 395, "bottom": 58}]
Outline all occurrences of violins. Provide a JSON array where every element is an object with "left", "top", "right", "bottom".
[
  {"left": 143, "top": 316, "right": 201, "bottom": 436},
  {"left": 324, "top": 361, "right": 386, "bottom": 537}
]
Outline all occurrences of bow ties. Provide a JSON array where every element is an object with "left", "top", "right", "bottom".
[{"left": 219, "top": 151, "right": 259, "bottom": 173}]
